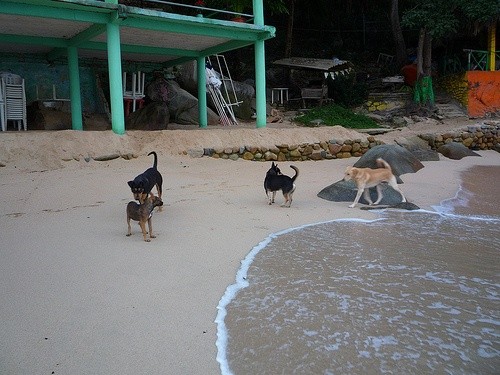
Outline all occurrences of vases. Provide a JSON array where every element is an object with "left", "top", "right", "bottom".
[{"left": 196, "top": 13, "right": 203, "bottom": 17}]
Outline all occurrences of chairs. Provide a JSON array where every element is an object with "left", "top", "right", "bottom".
[{"left": 0, "top": 73, "right": 27, "bottom": 131}]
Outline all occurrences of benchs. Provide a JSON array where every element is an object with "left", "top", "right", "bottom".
[{"left": 301, "top": 88, "right": 323, "bottom": 109}]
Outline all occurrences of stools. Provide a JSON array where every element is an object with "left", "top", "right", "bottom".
[
  {"left": 271, "top": 88, "right": 289, "bottom": 104},
  {"left": 122, "top": 71, "right": 145, "bottom": 117}
]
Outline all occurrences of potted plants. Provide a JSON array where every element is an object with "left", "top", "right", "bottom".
[{"left": 231, "top": 0, "right": 247, "bottom": 23}]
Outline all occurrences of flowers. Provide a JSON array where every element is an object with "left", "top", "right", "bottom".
[{"left": 195, "top": 0, "right": 205, "bottom": 13}]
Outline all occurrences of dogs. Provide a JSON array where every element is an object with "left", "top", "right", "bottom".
[
  {"left": 342, "top": 159, "right": 407, "bottom": 209},
  {"left": 126, "top": 195, "right": 164, "bottom": 242},
  {"left": 264, "top": 162, "right": 299, "bottom": 208},
  {"left": 127, "top": 151, "right": 163, "bottom": 211}
]
